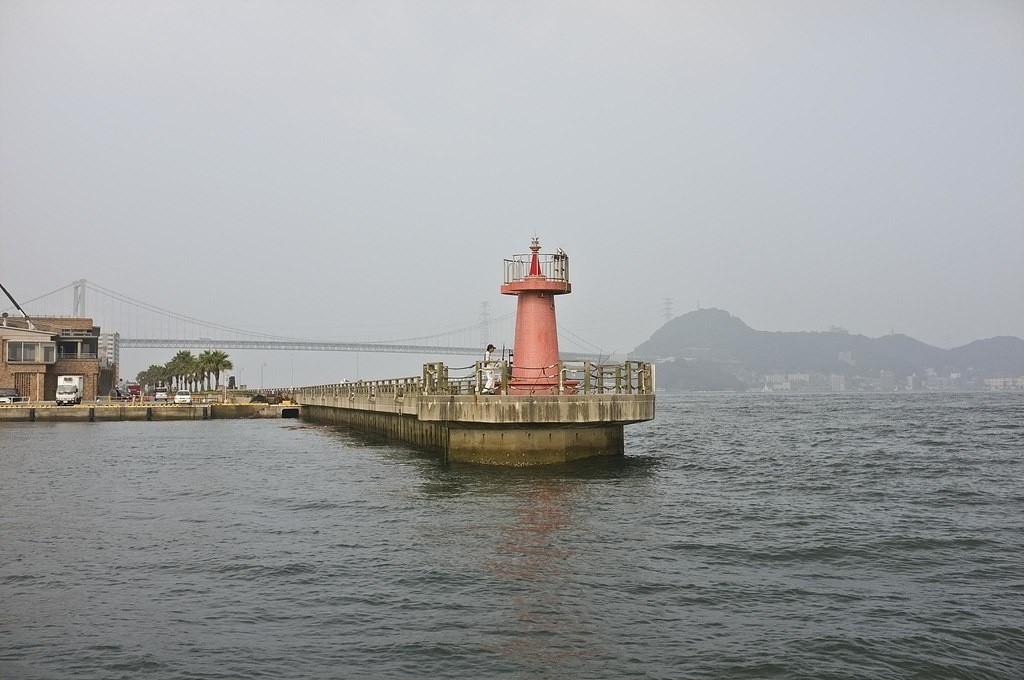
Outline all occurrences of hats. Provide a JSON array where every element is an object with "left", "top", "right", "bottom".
[{"left": 488, "top": 344, "right": 496, "bottom": 349}]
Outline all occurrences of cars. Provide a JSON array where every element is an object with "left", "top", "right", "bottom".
[{"left": 173, "top": 390, "right": 193, "bottom": 405}]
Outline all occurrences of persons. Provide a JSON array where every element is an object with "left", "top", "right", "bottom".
[{"left": 482, "top": 344, "right": 496, "bottom": 395}]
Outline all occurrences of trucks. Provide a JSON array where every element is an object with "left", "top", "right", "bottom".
[
  {"left": 53, "top": 375, "right": 83, "bottom": 406},
  {"left": 154, "top": 386, "right": 168, "bottom": 403}
]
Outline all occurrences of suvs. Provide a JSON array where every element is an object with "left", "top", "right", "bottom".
[{"left": 0, "top": 387, "right": 22, "bottom": 405}]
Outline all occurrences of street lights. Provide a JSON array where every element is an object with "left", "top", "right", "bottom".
[{"left": 261, "top": 363, "right": 266, "bottom": 389}]
